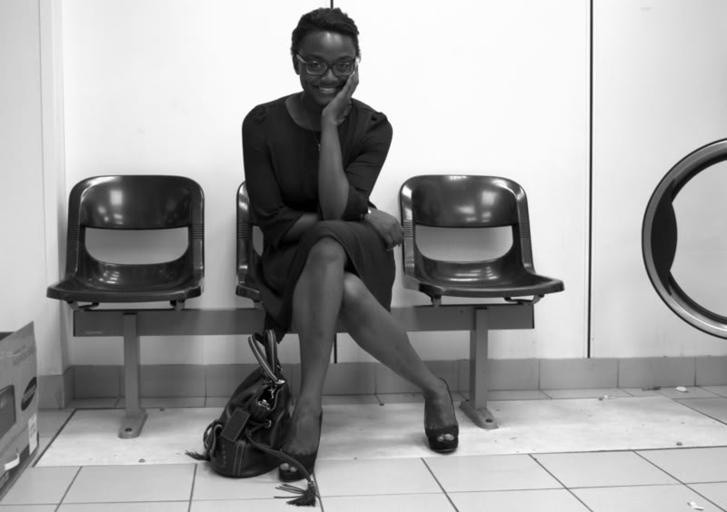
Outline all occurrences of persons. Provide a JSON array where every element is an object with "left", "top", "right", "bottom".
[{"left": 240, "top": 6, "right": 458, "bottom": 482}]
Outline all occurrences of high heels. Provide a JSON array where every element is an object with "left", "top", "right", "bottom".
[
  {"left": 423, "top": 376, "right": 459, "bottom": 453},
  {"left": 278, "top": 408, "right": 323, "bottom": 482}
]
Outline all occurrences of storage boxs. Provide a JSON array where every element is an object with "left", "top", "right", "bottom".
[{"left": 0, "top": 321, "right": 39, "bottom": 494}]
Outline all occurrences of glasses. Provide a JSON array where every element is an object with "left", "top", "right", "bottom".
[{"left": 296, "top": 55, "right": 355, "bottom": 76}]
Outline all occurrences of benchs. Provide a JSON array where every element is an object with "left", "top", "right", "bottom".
[{"left": 44, "top": 173, "right": 567, "bottom": 437}]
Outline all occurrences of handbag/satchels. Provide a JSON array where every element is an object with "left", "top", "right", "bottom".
[{"left": 186, "top": 328, "right": 317, "bottom": 507}]
